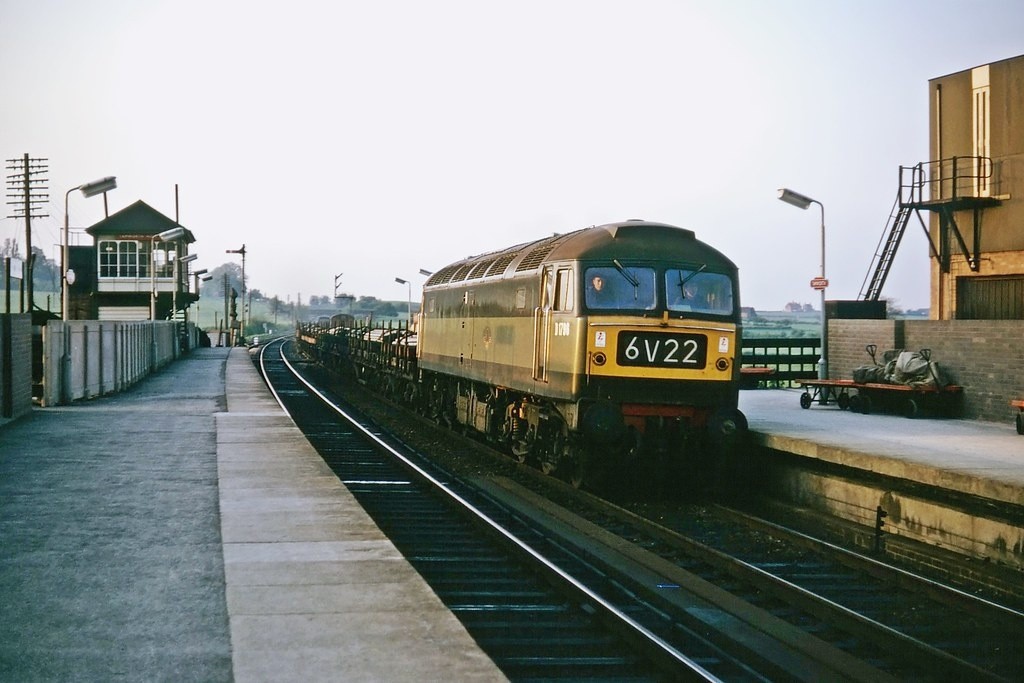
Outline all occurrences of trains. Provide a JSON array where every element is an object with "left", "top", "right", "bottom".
[{"left": 296, "top": 220, "right": 748, "bottom": 497}]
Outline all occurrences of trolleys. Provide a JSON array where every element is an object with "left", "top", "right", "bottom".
[{"left": 740, "top": 367, "right": 774, "bottom": 389}]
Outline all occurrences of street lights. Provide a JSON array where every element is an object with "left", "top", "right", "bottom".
[
  {"left": 59, "top": 175, "right": 118, "bottom": 403},
  {"left": 171, "top": 255, "right": 197, "bottom": 360},
  {"left": 774, "top": 187, "right": 829, "bottom": 404},
  {"left": 194, "top": 268, "right": 213, "bottom": 355},
  {"left": 149, "top": 226, "right": 183, "bottom": 374},
  {"left": 394, "top": 277, "right": 412, "bottom": 332}
]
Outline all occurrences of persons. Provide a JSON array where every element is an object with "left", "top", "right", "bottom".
[
  {"left": 672, "top": 278, "right": 712, "bottom": 310},
  {"left": 585, "top": 273, "right": 619, "bottom": 308}
]
